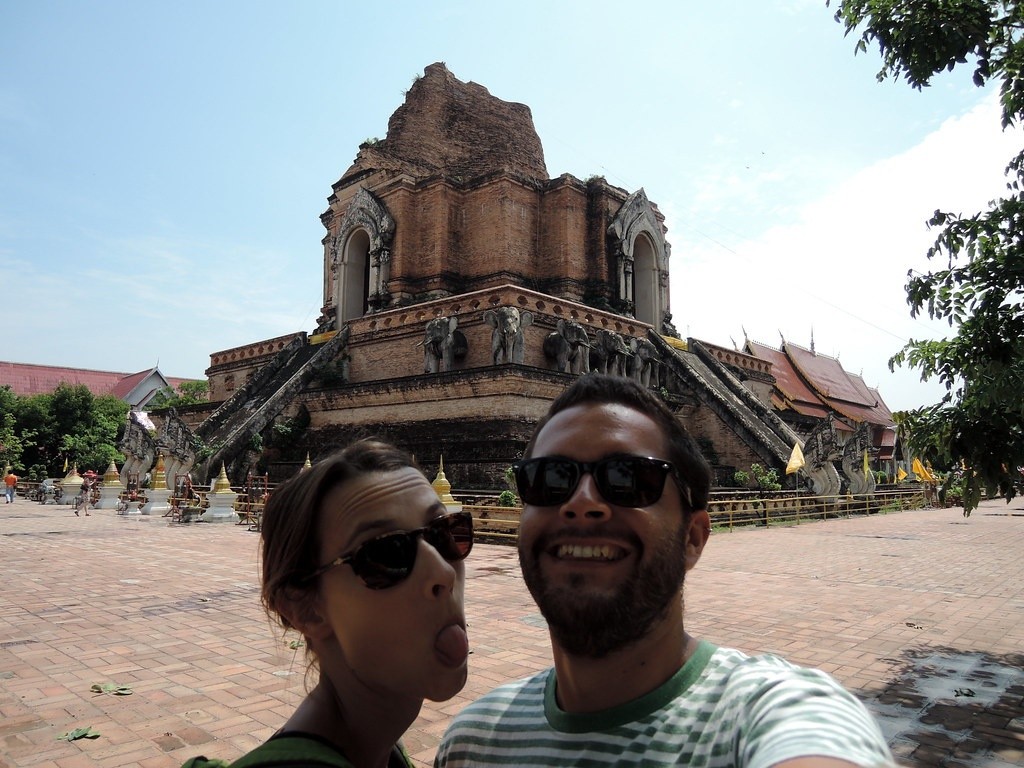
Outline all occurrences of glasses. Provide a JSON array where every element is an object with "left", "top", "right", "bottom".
[
  {"left": 299, "top": 512, "right": 474, "bottom": 590},
  {"left": 512, "top": 456, "right": 696, "bottom": 512}
]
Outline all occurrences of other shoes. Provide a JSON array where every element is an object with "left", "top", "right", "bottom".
[
  {"left": 75, "top": 511, "right": 79, "bottom": 516},
  {"left": 86, "top": 514, "right": 91, "bottom": 516}
]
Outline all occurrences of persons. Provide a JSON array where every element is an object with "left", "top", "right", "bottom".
[
  {"left": 433, "top": 374, "right": 895, "bottom": 768},
  {"left": 4, "top": 470, "right": 17, "bottom": 504},
  {"left": 74, "top": 470, "right": 97, "bottom": 516},
  {"left": 181, "top": 438, "right": 474, "bottom": 767}
]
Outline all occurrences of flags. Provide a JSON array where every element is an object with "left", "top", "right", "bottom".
[
  {"left": 864, "top": 448, "right": 869, "bottom": 482},
  {"left": 63, "top": 457, "right": 68, "bottom": 472},
  {"left": 912, "top": 457, "right": 932, "bottom": 480},
  {"left": 786, "top": 443, "right": 805, "bottom": 475},
  {"left": 898, "top": 466, "right": 908, "bottom": 482}
]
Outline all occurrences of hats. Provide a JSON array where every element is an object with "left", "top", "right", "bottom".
[{"left": 82, "top": 470, "right": 97, "bottom": 477}]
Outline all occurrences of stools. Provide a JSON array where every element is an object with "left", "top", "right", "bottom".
[{"left": 72, "top": 495, "right": 81, "bottom": 509}]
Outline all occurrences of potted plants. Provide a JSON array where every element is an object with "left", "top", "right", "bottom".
[{"left": 138, "top": 490, "right": 152, "bottom": 515}]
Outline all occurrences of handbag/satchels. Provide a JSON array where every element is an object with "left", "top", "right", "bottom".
[
  {"left": 80, "top": 485, "right": 89, "bottom": 491},
  {"left": 13, "top": 483, "right": 17, "bottom": 489}
]
